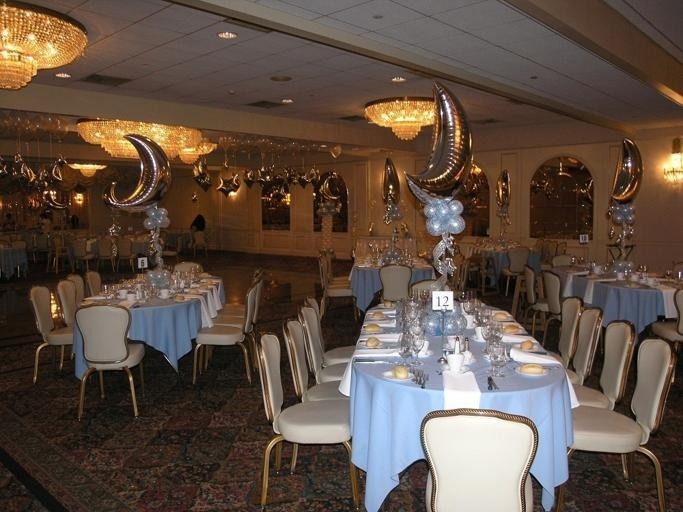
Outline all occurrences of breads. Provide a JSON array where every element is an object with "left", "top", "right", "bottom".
[
  {"left": 189, "top": 288, "right": 199, "bottom": 294},
  {"left": 648, "top": 272, "right": 657, "bottom": 279},
  {"left": 503, "top": 325, "right": 519, "bottom": 334},
  {"left": 521, "top": 340, "right": 534, "bottom": 350},
  {"left": 384, "top": 301, "right": 393, "bottom": 308},
  {"left": 199, "top": 273, "right": 208, "bottom": 277},
  {"left": 207, "top": 281, "right": 213, "bottom": 285},
  {"left": 520, "top": 364, "right": 543, "bottom": 374},
  {"left": 176, "top": 296, "right": 185, "bottom": 302},
  {"left": 494, "top": 312, "right": 508, "bottom": 322},
  {"left": 365, "top": 324, "right": 381, "bottom": 332},
  {"left": 366, "top": 337, "right": 380, "bottom": 347},
  {"left": 200, "top": 285, "right": 208, "bottom": 290},
  {"left": 630, "top": 283, "right": 640, "bottom": 288},
  {"left": 393, "top": 365, "right": 408, "bottom": 379}
]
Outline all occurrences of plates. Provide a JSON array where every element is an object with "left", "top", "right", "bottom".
[
  {"left": 379, "top": 303, "right": 394, "bottom": 309},
  {"left": 362, "top": 327, "right": 383, "bottom": 334},
  {"left": 513, "top": 343, "right": 543, "bottom": 352},
  {"left": 361, "top": 341, "right": 384, "bottom": 349},
  {"left": 513, "top": 367, "right": 549, "bottom": 378},
  {"left": 491, "top": 316, "right": 515, "bottom": 322},
  {"left": 366, "top": 314, "right": 389, "bottom": 321},
  {"left": 383, "top": 370, "right": 415, "bottom": 382},
  {"left": 502, "top": 326, "right": 525, "bottom": 337}
]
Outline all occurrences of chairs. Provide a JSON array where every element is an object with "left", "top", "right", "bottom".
[
  {"left": 459, "top": 245, "right": 475, "bottom": 288},
  {"left": 524, "top": 264, "right": 549, "bottom": 337},
  {"left": 55, "top": 235, "right": 74, "bottom": 274},
  {"left": 420, "top": 409, "right": 538, "bottom": 512},
  {"left": 411, "top": 279, "right": 450, "bottom": 299},
  {"left": 330, "top": 250, "right": 349, "bottom": 280},
  {"left": 55, "top": 278, "right": 77, "bottom": 361},
  {"left": 163, "top": 235, "right": 183, "bottom": 264},
  {"left": 192, "top": 230, "right": 208, "bottom": 258},
  {"left": 542, "top": 240, "right": 557, "bottom": 263},
  {"left": 116, "top": 239, "right": 136, "bottom": 273},
  {"left": 255, "top": 328, "right": 360, "bottom": 511},
  {"left": 222, "top": 270, "right": 260, "bottom": 310},
  {"left": 27, "top": 229, "right": 36, "bottom": 264},
  {"left": 304, "top": 295, "right": 356, "bottom": 368},
  {"left": 193, "top": 283, "right": 257, "bottom": 385},
  {"left": 379, "top": 264, "right": 412, "bottom": 303},
  {"left": 47, "top": 232, "right": 64, "bottom": 272},
  {"left": 318, "top": 254, "right": 355, "bottom": 310},
  {"left": 502, "top": 247, "right": 529, "bottom": 296},
  {"left": 652, "top": 288, "right": 683, "bottom": 387},
  {"left": 75, "top": 303, "right": 145, "bottom": 422},
  {"left": 133, "top": 241, "right": 144, "bottom": 257},
  {"left": 356, "top": 239, "right": 368, "bottom": 255},
  {"left": 463, "top": 247, "right": 481, "bottom": 290},
  {"left": 15, "top": 240, "right": 26, "bottom": 248},
  {"left": 283, "top": 317, "right": 350, "bottom": 475},
  {"left": 324, "top": 253, "right": 351, "bottom": 306},
  {"left": 96, "top": 238, "right": 115, "bottom": 272},
  {"left": 377, "top": 240, "right": 391, "bottom": 255},
  {"left": 552, "top": 255, "right": 570, "bottom": 267},
  {"left": 319, "top": 263, "right": 358, "bottom": 321},
  {"left": 160, "top": 231, "right": 167, "bottom": 250},
  {"left": 86, "top": 270, "right": 102, "bottom": 296},
  {"left": 29, "top": 285, "right": 92, "bottom": 384},
  {"left": 298, "top": 304, "right": 349, "bottom": 385},
  {"left": 205, "top": 280, "right": 263, "bottom": 372},
  {"left": 0, "top": 240, "right": 9, "bottom": 248},
  {"left": 566, "top": 306, "right": 603, "bottom": 385},
  {"left": 72, "top": 239, "right": 95, "bottom": 272},
  {"left": 171, "top": 232, "right": 189, "bottom": 248},
  {"left": 208, "top": 271, "right": 264, "bottom": 362},
  {"left": 557, "top": 335, "right": 676, "bottom": 512},
  {"left": 572, "top": 320, "right": 636, "bottom": 482},
  {"left": 168, "top": 233, "right": 186, "bottom": 259},
  {"left": 535, "top": 239, "right": 543, "bottom": 256},
  {"left": 673, "top": 262, "right": 683, "bottom": 278},
  {"left": 542, "top": 270, "right": 604, "bottom": 356},
  {"left": 479, "top": 251, "right": 500, "bottom": 296},
  {"left": 556, "top": 242, "right": 567, "bottom": 255},
  {"left": 549, "top": 296, "right": 584, "bottom": 369},
  {"left": 66, "top": 273, "right": 85, "bottom": 304}
]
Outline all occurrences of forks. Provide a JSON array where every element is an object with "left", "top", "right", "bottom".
[{"left": 421, "top": 372, "right": 430, "bottom": 388}]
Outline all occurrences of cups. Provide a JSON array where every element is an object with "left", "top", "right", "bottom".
[
  {"left": 490, "top": 325, "right": 504, "bottom": 350},
  {"left": 139, "top": 290, "right": 159, "bottom": 302},
  {"left": 401, "top": 296, "right": 424, "bottom": 334},
  {"left": 411, "top": 333, "right": 424, "bottom": 366},
  {"left": 116, "top": 289, "right": 128, "bottom": 300},
  {"left": 492, "top": 346, "right": 507, "bottom": 379},
  {"left": 480, "top": 323, "right": 493, "bottom": 353},
  {"left": 488, "top": 344, "right": 500, "bottom": 377},
  {"left": 666, "top": 268, "right": 672, "bottom": 284},
  {"left": 462, "top": 289, "right": 491, "bottom": 324},
  {"left": 127, "top": 294, "right": 139, "bottom": 304},
  {"left": 101, "top": 283, "right": 114, "bottom": 306},
  {"left": 396, "top": 335, "right": 412, "bottom": 368},
  {"left": 160, "top": 289, "right": 173, "bottom": 299},
  {"left": 447, "top": 354, "right": 465, "bottom": 373}
]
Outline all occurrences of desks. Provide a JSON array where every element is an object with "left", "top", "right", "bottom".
[
  {"left": 74, "top": 277, "right": 225, "bottom": 387},
  {"left": 351, "top": 304, "right": 573, "bottom": 512},
  {"left": 352, "top": 256, "right": 433, "bottom": 320},
  {"left": 549, "top": 266, "right": 683, "bottom": 345},
  {"left": 65, "top": 234, "right": 150, "bottom": 255}
]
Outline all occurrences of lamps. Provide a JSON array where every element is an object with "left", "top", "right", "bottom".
[
  {"left": 0, "top": 0, "right": 89, "bottom": 91},
  {"left": 199, "top": 142, "right": 218, "bottom": 155},
  {"left": 180, "top": 148, "right": 198, "bottom": 164},
  {"left": 76, "top": 120, "right": 202, "bottom": 159},
  {"left": 664, "top": 138, "right": 683, "bottom": 183},
  {"left": 69, "top": 163, "right": 108, "bottom": 177},
  {"left": 364, "top": 97, "right": 435, "bottom": 142}
]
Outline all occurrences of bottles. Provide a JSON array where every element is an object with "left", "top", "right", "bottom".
[
  {"left": 373, "top": 311, "right": 384, "bottom": 319},
  {"left": 463, "top": 337, "right": 472, "bottom": 362},
  {"left": 455, "top": 337, "right": 460, "bottom": 354},
  {"left": 136, "top": 288, "right": 139, "bottom": 299}
]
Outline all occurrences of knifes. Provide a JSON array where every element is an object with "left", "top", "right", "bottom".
[{"left": 486, "top": 374, "right": 492, "bottom": 391}]
